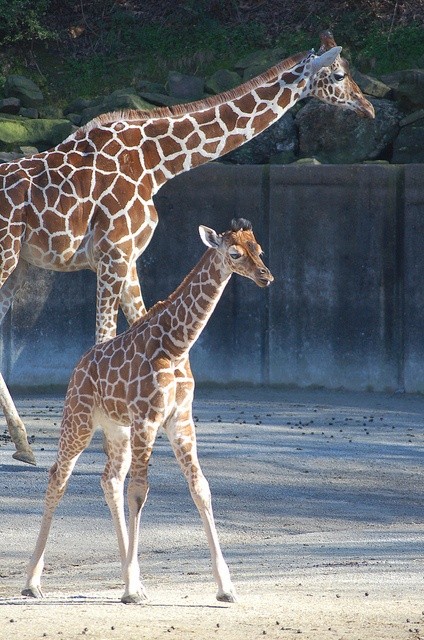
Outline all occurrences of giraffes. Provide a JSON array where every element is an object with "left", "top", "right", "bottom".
[
  {"left": 20, "top": 216, "right": 274, "bottom": 605},
  {"left": 0, "top": 30, "right": 378, "bottom": 469}
]
